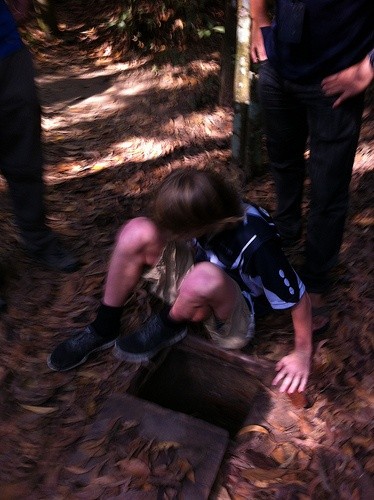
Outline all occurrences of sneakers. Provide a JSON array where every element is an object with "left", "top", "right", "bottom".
[
  {"left": 114, "top": 321, "right": 189, "bottom": 362},
  {"left": 46, "top": 318, "right": 123, "bottom": 372}
]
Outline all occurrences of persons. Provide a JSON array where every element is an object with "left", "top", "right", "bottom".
[
  {"left": 247, "top": 0, "right": 374, "bottom": 323},
  {"left": 47, "top": 167, "right": 313, "bottom": 395},
  {"left": 0, "top": 0, "right": 82, "bottom": 278}
]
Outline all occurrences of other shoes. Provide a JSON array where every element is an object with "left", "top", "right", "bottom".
[{"left": 311, "top": 305, "right": 332, "bottom": 335}]
